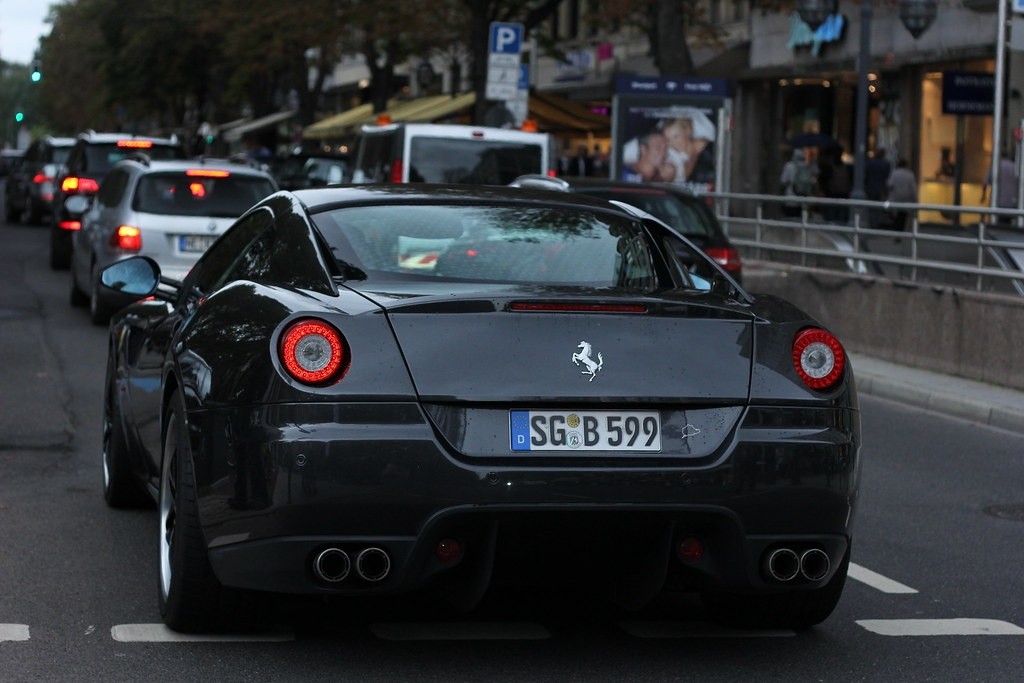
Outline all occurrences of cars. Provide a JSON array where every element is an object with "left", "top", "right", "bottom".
[
  {"left": 506, "top": 170, "right": 744, "bottom": 282},
  {"left": 66, "top": 158, "right": 283, "bottom": 326},
  {"left": 47, "top": 126, "right": 184, "bottom": 267},
  {"left": 95, "top": 181, "right": 863, "bottom": 632},
  {"left": 4, "top": 133, "right": 78, "bottom": 226}
]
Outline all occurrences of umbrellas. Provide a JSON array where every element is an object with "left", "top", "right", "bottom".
[{"left": 785, "top": 131, "right": 839, "bottom": 147}]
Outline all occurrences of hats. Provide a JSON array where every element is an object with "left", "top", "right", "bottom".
[{"left": 794, "top": 148, "right": 804, "bottom": 160}]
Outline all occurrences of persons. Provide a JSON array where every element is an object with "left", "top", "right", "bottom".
[
  {"left": 934, "top": 147, "right": 955, "bottom": 178},
  {"left": 556, "top": 141, "right": 611, "bottom": 178},
  {"left": 620, "top": 105, "right": 718, "bottom": 196},
  {"left": 776, "top": 147, "right": 920, "bottom": 244},
  {"left": 980, "top": 149, "right": 1019, "bottom": 225}
]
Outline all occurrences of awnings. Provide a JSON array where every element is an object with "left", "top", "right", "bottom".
[
  {"left": 302, "top": 90, "right": 476, "bottom": 140},
  {"left": 199, "top": 107, "right": 302, "bottom": 144}
]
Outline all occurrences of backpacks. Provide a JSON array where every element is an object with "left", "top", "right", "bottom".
[{"left": 793, "top": 160, "right": 812, "bottom": 196}]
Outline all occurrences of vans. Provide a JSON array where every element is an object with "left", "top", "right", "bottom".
[{"left": 356, "top": 121, "right": 555, "bottom": 195}]
